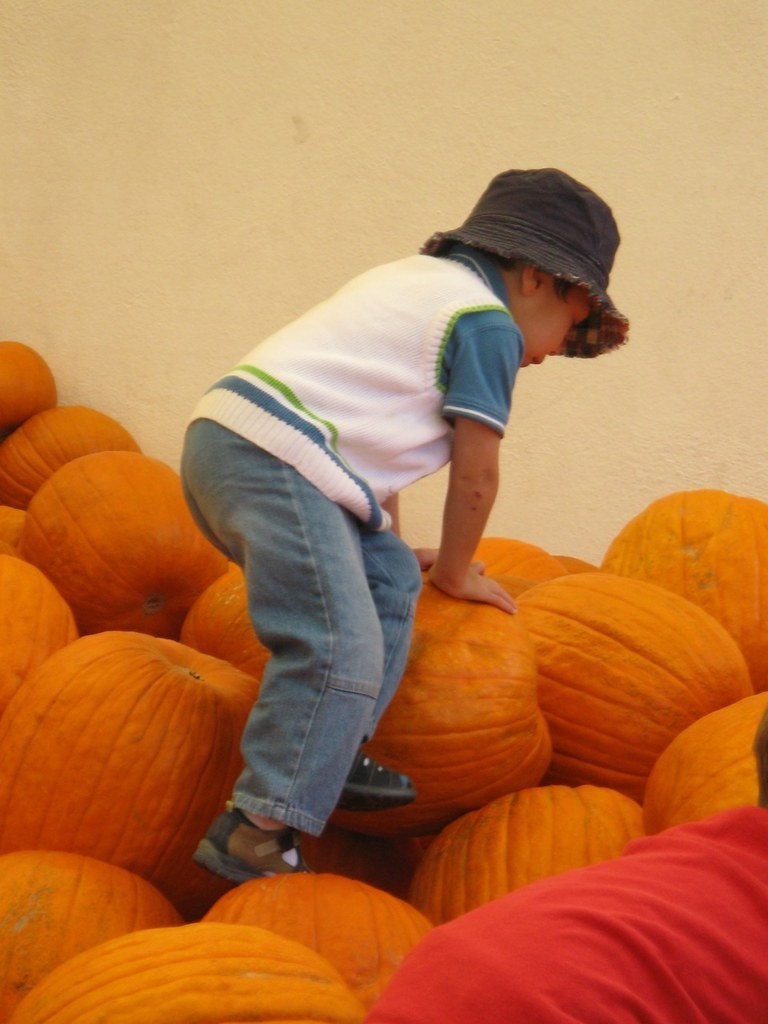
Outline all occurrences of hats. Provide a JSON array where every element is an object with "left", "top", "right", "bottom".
[{"left": 420, "top": 167, "right": 628, "bottom": 360}]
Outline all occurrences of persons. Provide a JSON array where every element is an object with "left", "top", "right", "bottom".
[
  {"left": 180, "top": 168, "right": 631, "bottom": 879},
  {"left": 360, "top": 710, "right": 768, "bottom": 1024}
]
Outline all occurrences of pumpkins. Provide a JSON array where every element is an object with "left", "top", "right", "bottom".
[{"left": 0, "top": 335, "right": 768, "bottom": 1024}]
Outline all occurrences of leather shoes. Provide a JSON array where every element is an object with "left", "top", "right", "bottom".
[
  {"left": 338, "top": 750, "right": 416, "bottom": 810},
  {"left": 193, "top": 801, "right": 317, "bottom": 884}
]
